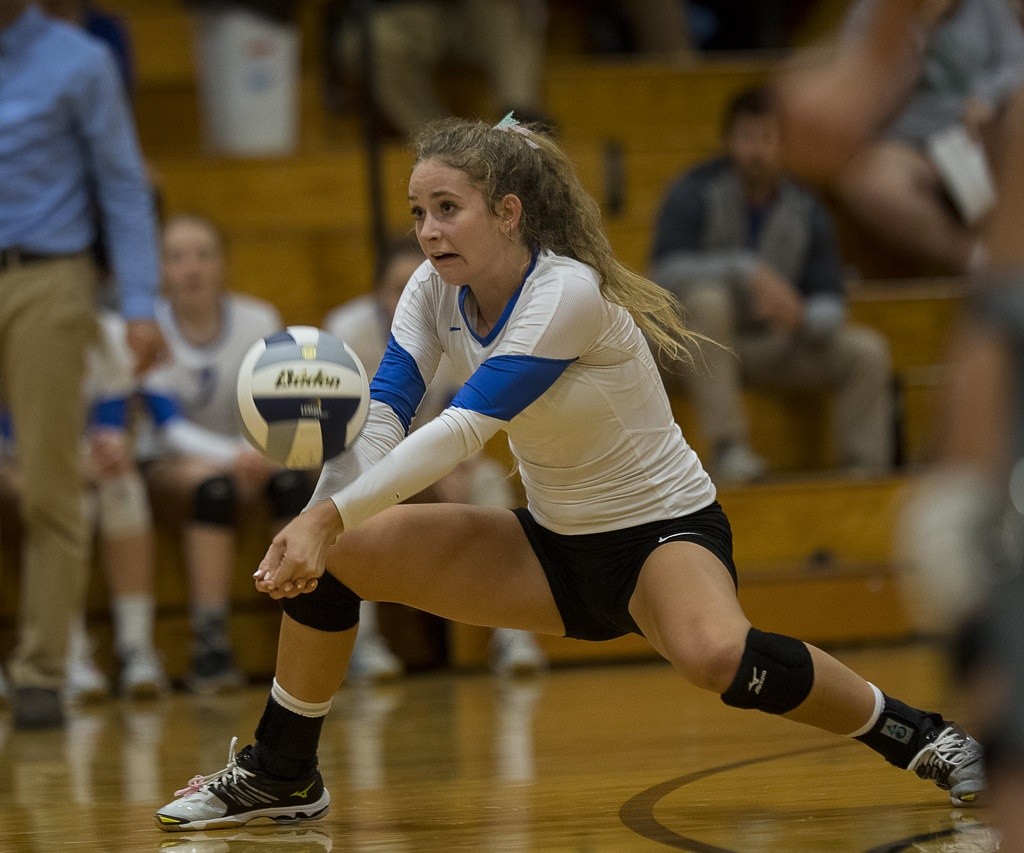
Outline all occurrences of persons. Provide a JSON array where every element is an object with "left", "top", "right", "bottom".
[
  {"left": 153, "top": 113, "right": 994, "bottom": 831},
  {"left": 0, "top": 0, "right": 1024, "bottom": 746}
]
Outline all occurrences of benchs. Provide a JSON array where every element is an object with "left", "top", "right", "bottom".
[{"left": 83, "top": 69, "right": 975, "bottom": 682}]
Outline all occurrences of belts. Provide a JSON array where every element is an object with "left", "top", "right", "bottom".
[{"left": 0, "top": 245, "right": 50, "bottom": 273}]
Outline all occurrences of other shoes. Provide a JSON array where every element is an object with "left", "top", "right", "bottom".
[
  {"left": 184, "top": 649, "right": 243, "bottom": 693},
  {"left": 350, "top": 631, "right": 404, "bottom": 681},
  {"left": 60, "top": 666, "right": 111, "bottom": 704},
  {"left": 720, "top": 446, "right": 769, "bottom": 483},
  {"left": 120, "top": 662, "right": 173, "bottom": 701},
  {"left": 491, "top": 626, "right": 550, "bottom": 678},
  {"left": 11, "top": 687, "right": 67, "bottom": 730}
]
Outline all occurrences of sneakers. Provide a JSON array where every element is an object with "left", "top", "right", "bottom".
[
  {"left": 152, "top": 736, "right": 331, "bottom": 831},
  {"left": 905, "top": 719, "right": 994, "bottom": 809}
]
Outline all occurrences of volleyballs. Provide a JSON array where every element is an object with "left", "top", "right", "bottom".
[{"left": 235, "top": 325, "right": 370, "bottom": 469}]
